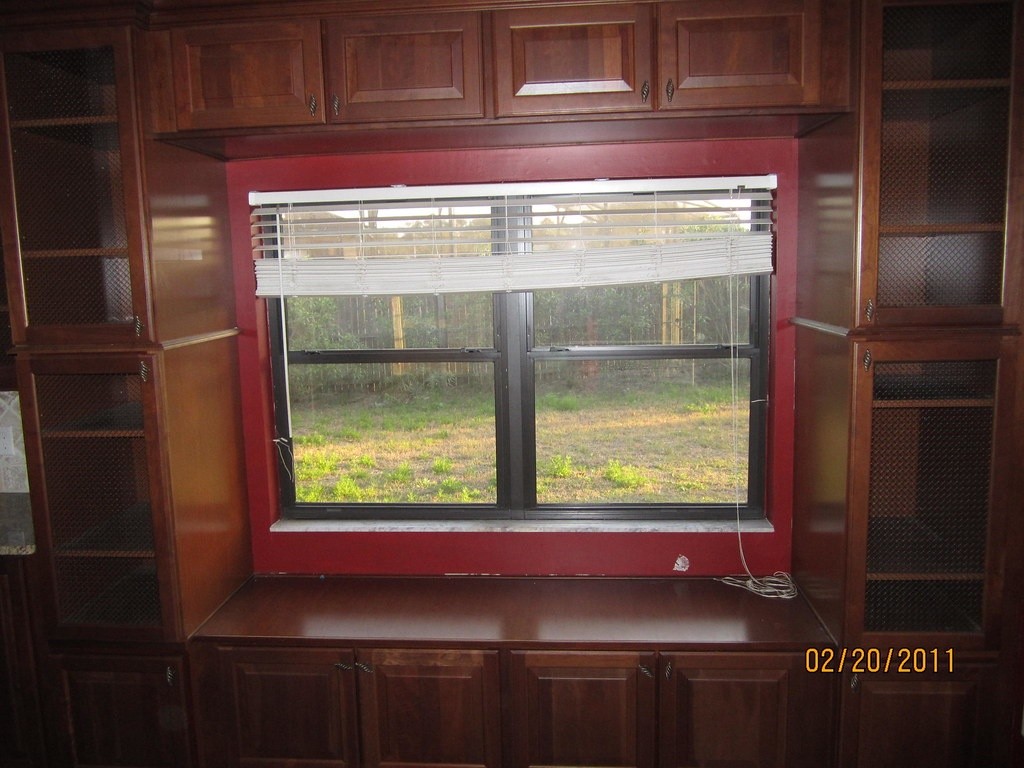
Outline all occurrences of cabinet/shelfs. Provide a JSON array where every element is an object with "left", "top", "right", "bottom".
[{"left": 0, "top": 0, "right": 1024, "bottom": 768}]
